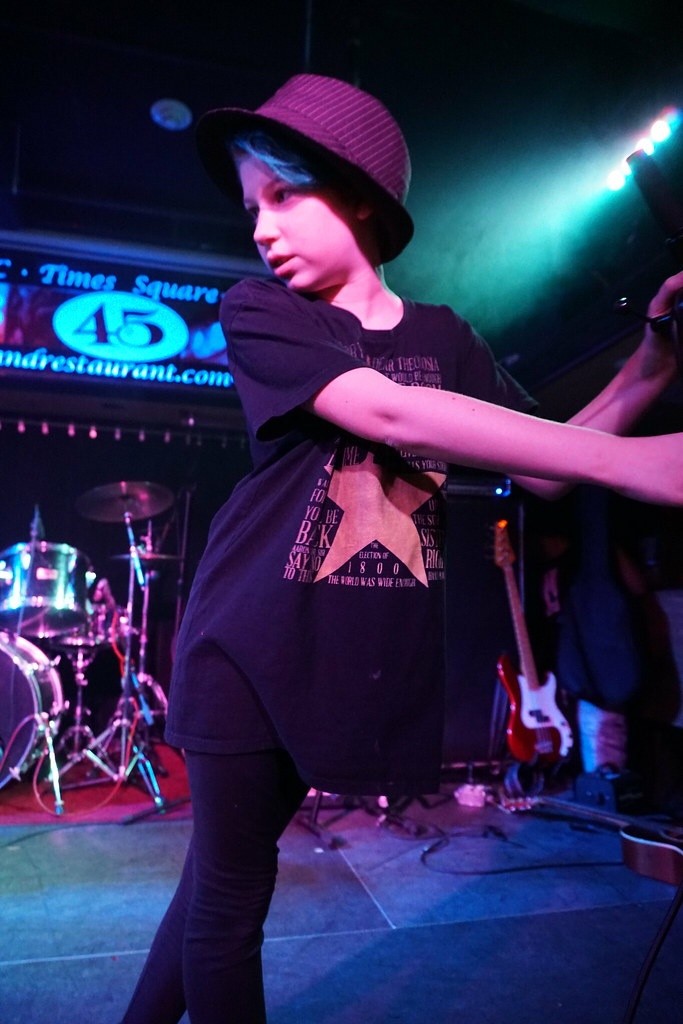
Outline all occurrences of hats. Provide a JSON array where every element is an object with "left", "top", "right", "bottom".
[{"left": 203, "top": 73, "right": 413, "bottom": 267}]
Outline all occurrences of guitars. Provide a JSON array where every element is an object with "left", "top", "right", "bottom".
[
  {"left": 622, "top": 822, "right": 681, "bottom": 887},
  {"left": 493, "top": 518, "right": 574, "bottom": 764}
]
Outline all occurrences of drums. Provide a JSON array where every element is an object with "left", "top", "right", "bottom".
[
  {"left": 1, "top": 540, "right": 91, "bottom": 639},
  {"left": 0, "top": 629, "right": 58, "bottom": 794},
  {"left": 51, "top": 602, "right": 131, "bottom": 649}
]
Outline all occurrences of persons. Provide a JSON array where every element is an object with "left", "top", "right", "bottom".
[
  {"left": 115, "top": 74, "right": 683, "bottom": 1024},
  {"left": 529, "top": 534, "right": 657, "bottom": 813}
]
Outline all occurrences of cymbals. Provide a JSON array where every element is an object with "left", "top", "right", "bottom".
[
  {"left": 73, "top": 477, "right": 174, "bottom": 523},
  {"left": 112, "top": 550, "right": 185, "bottom": 570}
]
